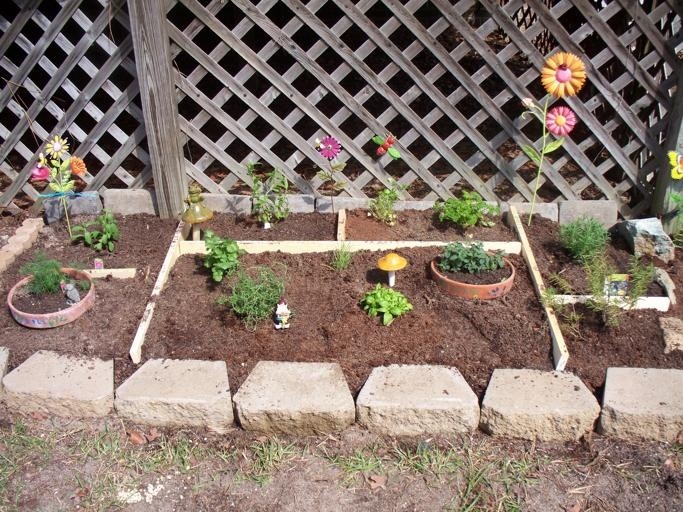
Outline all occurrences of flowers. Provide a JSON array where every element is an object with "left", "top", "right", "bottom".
[
  {"left": 30, "top": 133, "right": 88, "bottom": 242},
  {"left": 520, "top": 49, "right": 586, "bottom": 227},
  {"left": 315, "top": 136, "right": 348, "bottom": 219}
]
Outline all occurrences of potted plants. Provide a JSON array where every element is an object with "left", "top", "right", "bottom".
[
  {"left": 429, "top": 240, "right": 517, "bottom": 301},
  {"left": 7, "top": 249, "right": 94, "bottom": 330}
]
